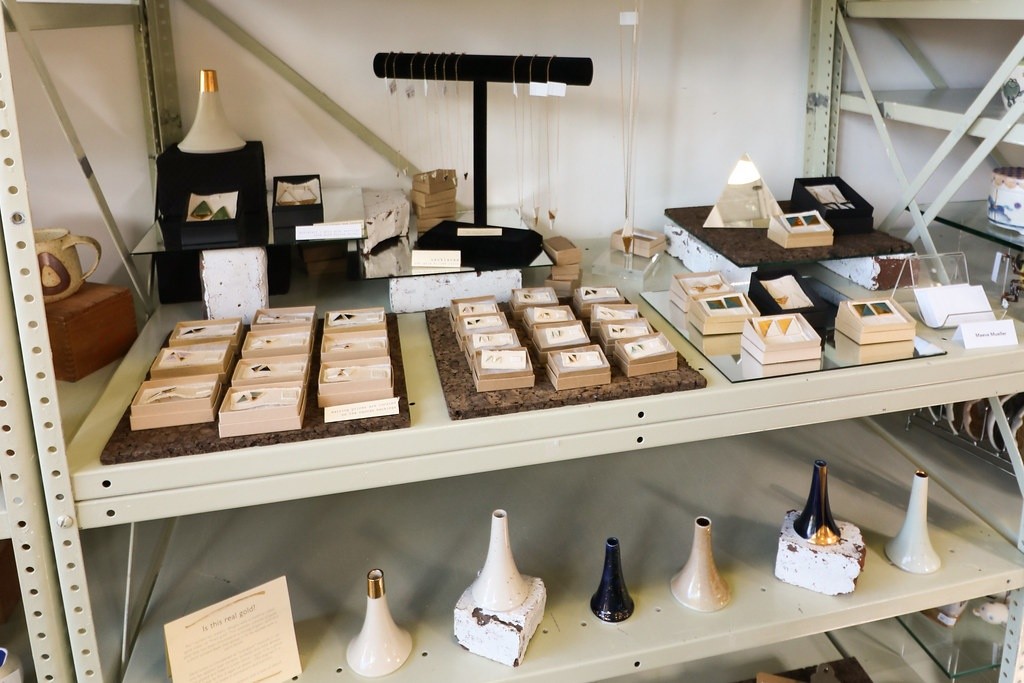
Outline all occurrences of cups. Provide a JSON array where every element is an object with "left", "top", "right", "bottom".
[{"left": 33, "top": 228, "right": 102, "bottom": 302}]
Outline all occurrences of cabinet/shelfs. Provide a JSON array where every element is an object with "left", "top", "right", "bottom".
[
  {"left": 0, "top": 0, "right": 164, "bottom": 683},
  {"left": 809, "top": 0, "right": 1024, "bottom": 683},
  {"left": 0, "top": 0, "right": 1024, "bottom": 683}
]
